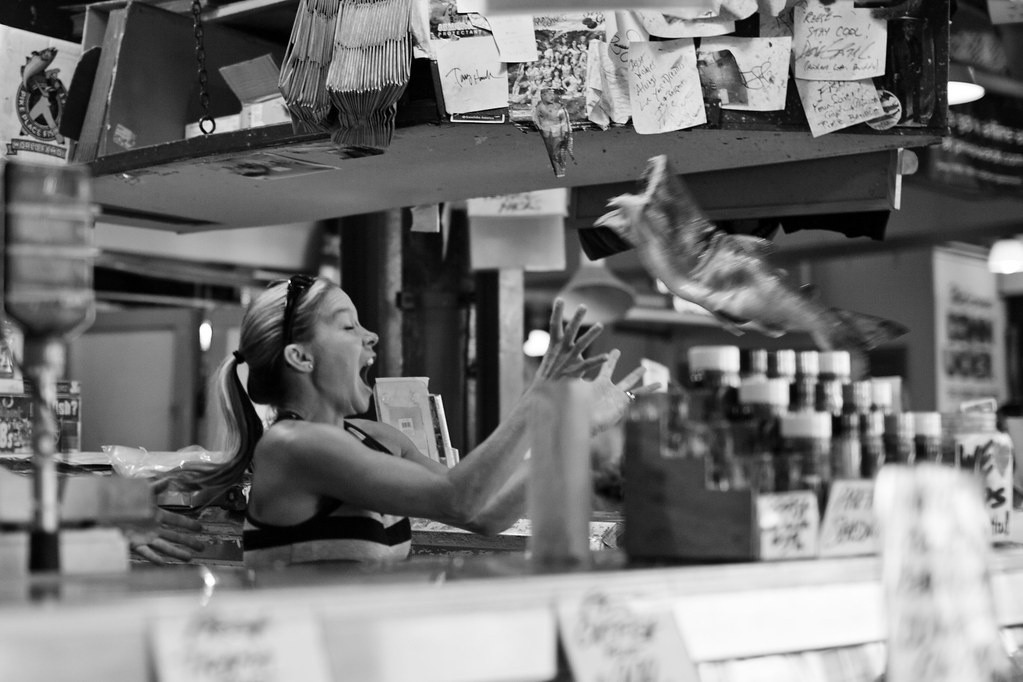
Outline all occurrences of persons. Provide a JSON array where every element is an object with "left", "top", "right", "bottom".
[{"left": 184, "top": 272, "right": 662, "bottom": 568}]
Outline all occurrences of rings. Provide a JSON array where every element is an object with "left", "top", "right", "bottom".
[{"left": 624, "top": 390, "right": 635, "bottom": 400}]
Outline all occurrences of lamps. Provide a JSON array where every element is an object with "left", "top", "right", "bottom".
[{"left": 948, "top": 63, "right": 986, "bottom": 106}]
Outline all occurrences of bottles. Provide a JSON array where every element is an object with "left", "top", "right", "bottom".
[{"left": 686, "top": 345, "right": 1015, "bottom": 548}]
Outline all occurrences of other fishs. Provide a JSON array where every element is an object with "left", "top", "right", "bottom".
[{"left": 595, "top": 153, "right": 915, "bottom": 353}]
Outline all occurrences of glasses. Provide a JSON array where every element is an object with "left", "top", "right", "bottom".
[{"left": 283, "top": 275, "right": 317, "bottom": 348}]
